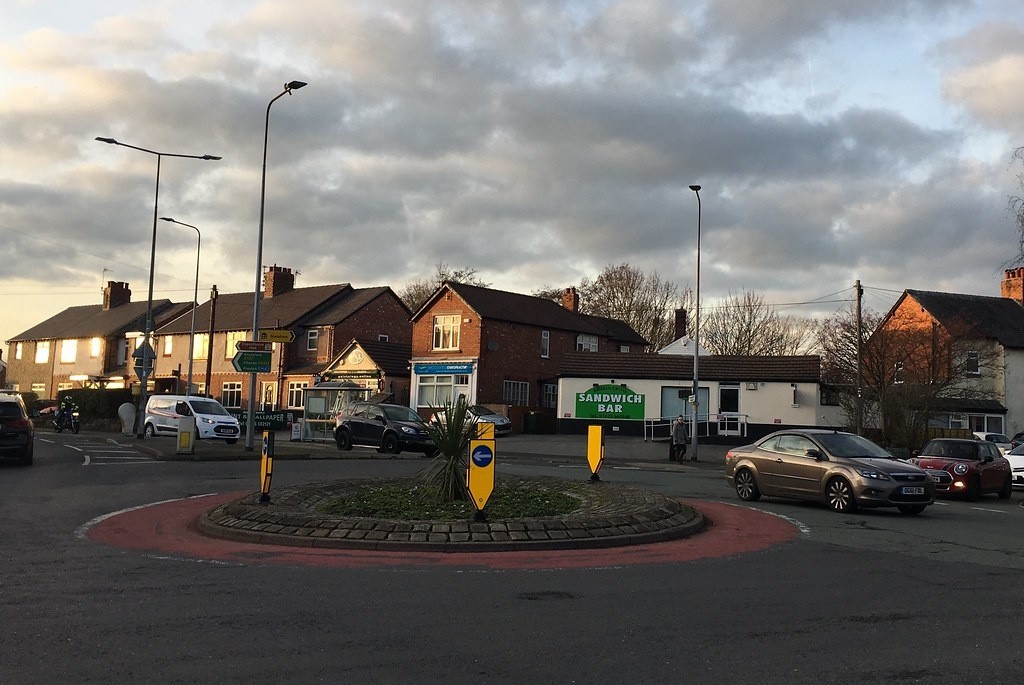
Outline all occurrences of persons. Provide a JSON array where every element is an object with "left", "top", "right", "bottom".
[
  {"left": 57, "top": 395, "right": 79, "bottom": 434},
  {"left": 672, "top": 415, "right": 689, "bottom": 462}
]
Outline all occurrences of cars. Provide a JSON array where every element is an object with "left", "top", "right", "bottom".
[
  {"left": 907, "top": 437, "right": 1013, "bottom": 502},
  {"left": 722, "top": 429, "right": 936, "bottom": 516},
  {"left": 1002, "top": 442, "right": 1024, "bottom": 486},
  {"left": 428, "top": 403, "right": 513, "bottom": 438},
  {"left": 974, "top": 431, "right": 1024, "bottom": 455}
]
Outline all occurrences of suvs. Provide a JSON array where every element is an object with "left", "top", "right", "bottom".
[
  {"left": 0, "top": 395, "right": 41, "bottom": 466},
  {"left": 1, "top": 388, "right": 29, "bottom": 415},
  {"left": 331, "top": 400, "right": 441, "bottom": 459}
]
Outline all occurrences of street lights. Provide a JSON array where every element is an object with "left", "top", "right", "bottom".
[
  {"left": 244, "top": 80, "right": 309, "bottom": 451},
  {"left": 94, "top": 136, "right": 223, "bottom": 402},
  {"left": 159, "top": 215, "right": 201, "bottom": 397},
  {"left": 689, "top": 183, "right": 702, "bottom": 463}
]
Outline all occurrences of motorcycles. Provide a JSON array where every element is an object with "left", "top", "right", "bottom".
[{"left": 50, "top": 405, "right": 81, "bottom": 434}]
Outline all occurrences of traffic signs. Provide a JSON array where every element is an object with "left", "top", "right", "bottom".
[
  {"left": 231, "top": 351, "right": 273, "bottom": 373},
  {"left": 257, "top": 330, "right": 296, "bottom": 343},
  {"left": 235, "top": 340, "right": 273, "bottom": 352}
]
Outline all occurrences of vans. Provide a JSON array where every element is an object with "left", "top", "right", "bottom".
[{"left": 144, "top": 395, "right": 241, "bottom": 444}]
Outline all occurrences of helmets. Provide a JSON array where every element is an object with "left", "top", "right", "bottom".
[{"left": 65, "top": 396, "right": 72, "bottom": 403}]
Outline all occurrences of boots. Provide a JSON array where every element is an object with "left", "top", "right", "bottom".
[
  {"left": 679, "top": 452, "right": 685, "bottom": 465},
  {"left": 676, "top": 452, "right": 681, "bottom": 465}
]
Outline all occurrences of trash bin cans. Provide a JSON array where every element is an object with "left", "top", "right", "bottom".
[{"left": 526, "top": 411, "right": 544, "bottom": 433}]
